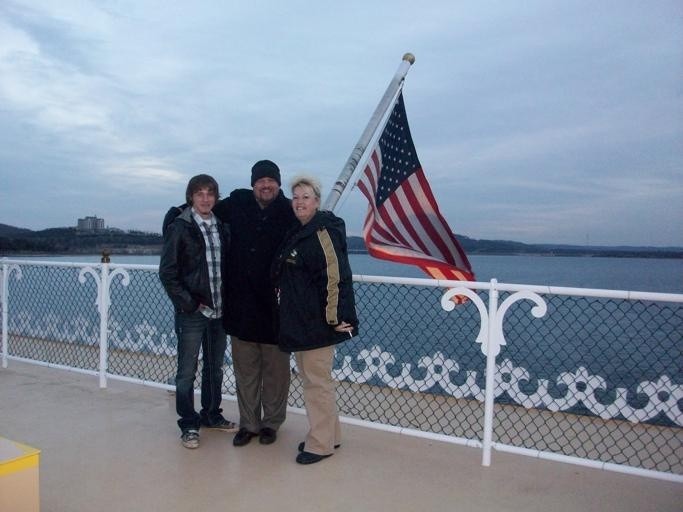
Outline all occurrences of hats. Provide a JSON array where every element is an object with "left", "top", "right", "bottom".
[{"left": 251, "top": 160, "right": 281, "bottom": 188}]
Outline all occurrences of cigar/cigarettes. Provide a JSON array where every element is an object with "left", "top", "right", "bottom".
[{"left": 348, "top": 331, "right": 352, "bottom": 338}]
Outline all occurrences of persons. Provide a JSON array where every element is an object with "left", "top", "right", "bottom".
[
  {"left": 163, "top": 160, "right": 297, "bottom": 445},
  {"left": 160, "top": 175, "right": 241, "bottom": 448},
  {"left": 279, "top": 180, "right": 357, "bottom": 465}
]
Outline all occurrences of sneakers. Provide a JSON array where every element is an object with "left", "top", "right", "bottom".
[
  {"left": 233, "top": 427, "right": 258, "bottom": 447},
  {"left": 297, "top": 441, "right": 341, "bottom": 452},
  {"left": 204, "top": 420, "right": 240, "bottom": 432},
  {"left": 258, "top": 428, "right": 278, "bottom": 444},
  {"left": 296, "top": 449, "right": 334, "bottom": 466},
  {"left": 181, "top": 427, "right": 201, "bottom": 450}
]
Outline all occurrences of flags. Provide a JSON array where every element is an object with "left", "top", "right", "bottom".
[{"left": 355, "top": 90, "right": 479, "bottom": 304}]
